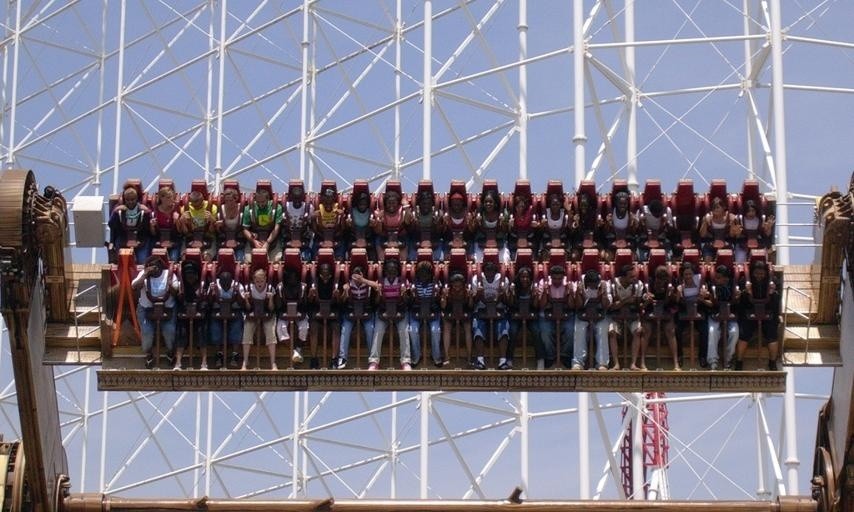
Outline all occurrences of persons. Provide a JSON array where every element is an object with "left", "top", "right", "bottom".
[{"left": 109, "top": 188, "right": 786, "bottom": 370}]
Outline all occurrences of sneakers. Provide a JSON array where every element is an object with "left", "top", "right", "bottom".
[{"left": 144, "top": 351, "right": 781, "bottom": 375}]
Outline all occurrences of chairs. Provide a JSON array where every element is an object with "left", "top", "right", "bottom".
[{"left": 100, "top": 178, "right": 784, "bottom": 358}]
